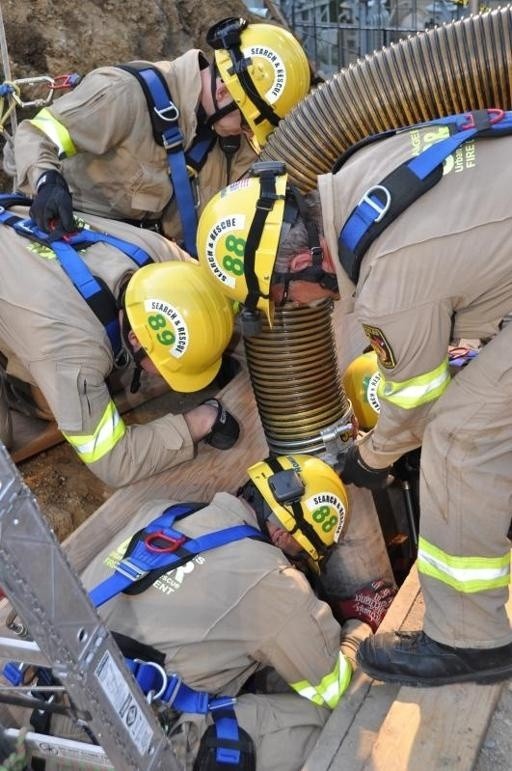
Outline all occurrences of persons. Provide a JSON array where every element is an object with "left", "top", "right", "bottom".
[
  {"left": 196, "top": 111, "right": 512, "bottom": 687},
  {"left": 1, "top": 194, "right": 239, "bottom": 488},
  {"left": 1, "top": 456, "right": 398, "bottom": 770},
  {"left": 0, "top": 18, "right": 311, "bottom": 233}
]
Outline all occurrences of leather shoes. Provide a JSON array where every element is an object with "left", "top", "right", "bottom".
[{"left": 354, "top": 627, "right": 512, "bottom": 686}]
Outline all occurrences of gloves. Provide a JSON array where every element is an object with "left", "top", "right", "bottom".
[
  {"left": 339, "top": 580, "right": 398, "bottom": 633},
  {"left": 339, "top": 445, "right": 394, "bottom": 494},
  {"left": 27, "top": 169, "right": 78, "bottom": 235},
  {"left": 198, "top": 397, "right": 240, "bottom": 451}
]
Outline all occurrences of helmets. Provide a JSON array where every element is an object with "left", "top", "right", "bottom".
[
  {"left": 206, "top": 16, "right": 310, "bottom": 146},
  {"left": 246, "top": 455, "right": 349, "bottom": 578},
  {"left": 196, "top": 162, "right": 289, "bottom": 331},
  {"left": 124, "top": 260, "right": 235, "bottom": 395},
  {"left": 341, "top": 345, "right": 384, "bottom": 434}
]
